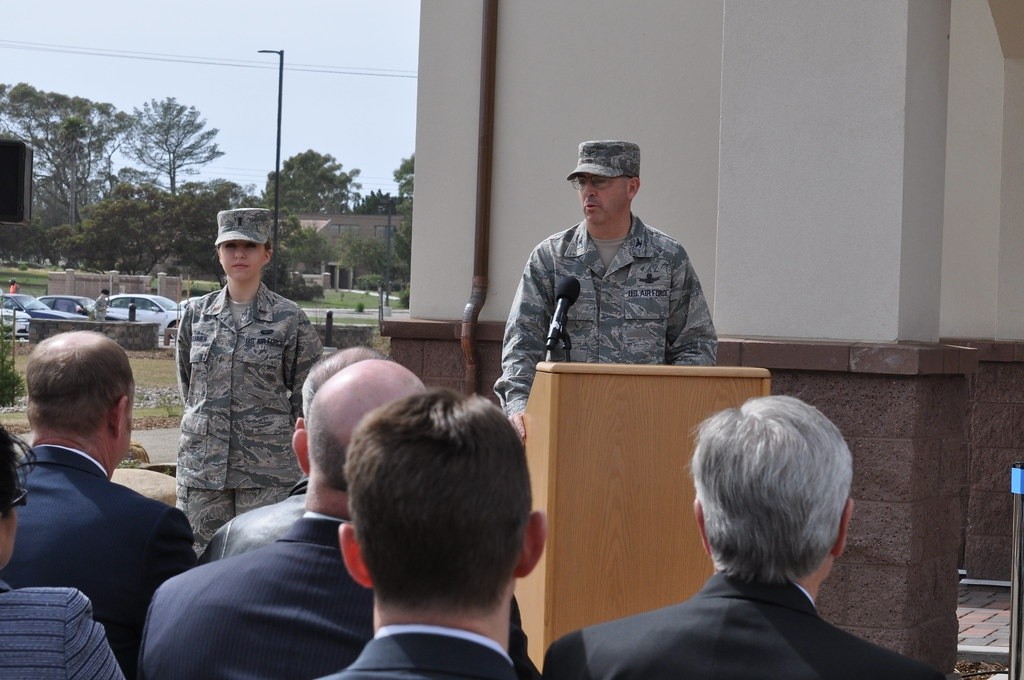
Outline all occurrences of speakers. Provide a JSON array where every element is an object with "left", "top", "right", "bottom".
[{"left": 0, "top": 140, "right": 33, "bottom": 226}]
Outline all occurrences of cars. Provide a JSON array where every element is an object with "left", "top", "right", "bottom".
[
  {"left": 24, "top": 294, "right": 96, "bottom": 325},
  {"left": 0, "top": 292, "right": 90, "bottom": 321},
  {"left": 85, "top": 293, "right": 188, "bottom": 341},
  {"left": 179, "top": 296, "right": 201, "bottom": 310},
  {"left": 0, "top": 308, "right": 32, "bottom": 340}
]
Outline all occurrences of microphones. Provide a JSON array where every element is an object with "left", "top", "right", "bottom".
[{"left": 546, "top": 277, "right": 581, "bottom": 350}]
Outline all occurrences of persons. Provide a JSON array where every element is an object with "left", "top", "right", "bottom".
[
  {"left": 1, "top": 427, "right": 126, "bottom": 680},
  {"left": 135, "top": 359, "right": 541, "bottom": 680},
  {"left": 494, "top": 140, "right": 718, "bottom": 448},
  {"left": 314, "top": 390, "right": 548, "bottom": 680},
  {"left": 197, "top": 346, "right": 398, "bottom": 565},
  {"left": 9, "top": 278, "right": 20, "bottom": 294},
  {"left": 175, "top": 208, "right": 323, "bottom": 557},
  {"left": 1, "top": 330, "right": 197, "bottom": 680},
  {"left": 541, "top": 394, "right": 944, "bottom": 680},
  {"left": 93, "top": 289, "right": 110, "bottom": 321}
]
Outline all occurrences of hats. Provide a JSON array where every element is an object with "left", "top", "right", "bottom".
[
  {"left": 101, "top": 289, "right": 109, "bottom": 295},
  {"left": 9, "top": 278, "right": 16, "bottom": 283},
  {"left": 214, "top": 208, "right": 271, "bottom": 245},
  {"left": 567, "top": 140, "right": 640, "bottom": 179}
]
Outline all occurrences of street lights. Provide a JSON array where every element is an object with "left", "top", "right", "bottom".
[{"left": 257, "top": 49, "right": 285, "bottom": 291}]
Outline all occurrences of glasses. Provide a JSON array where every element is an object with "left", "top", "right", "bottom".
[
  {"left": 570, "top": 172, "right": 612, "bottom": 189},
  {"left": 6, "top": 485, "right": 28, "bottom": 510}
]
